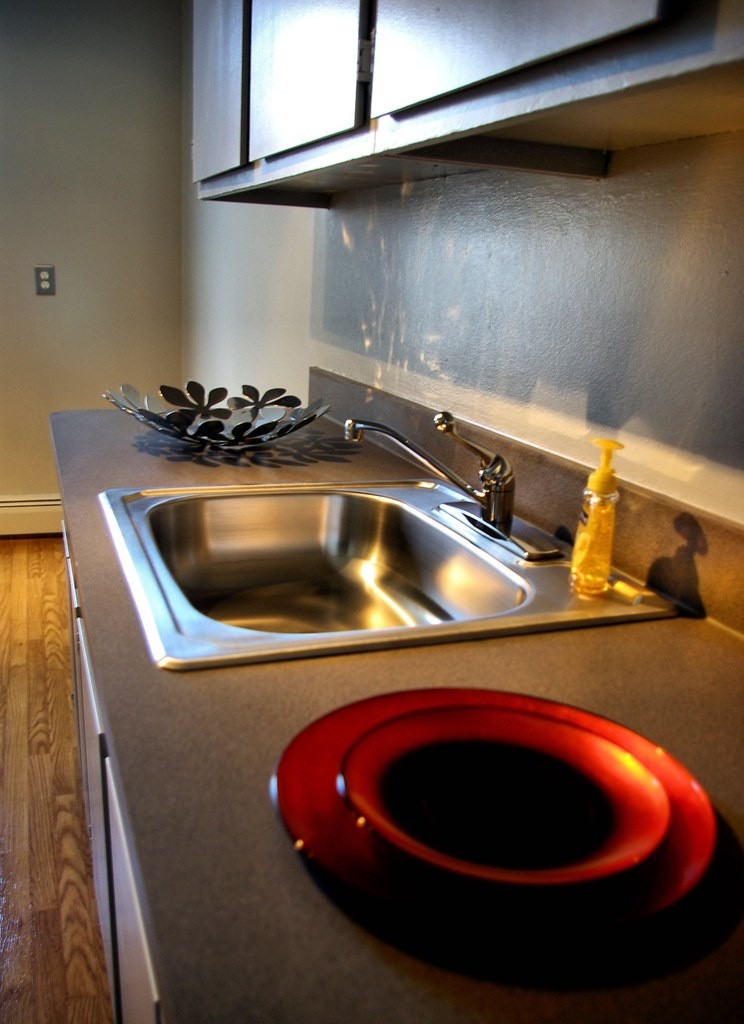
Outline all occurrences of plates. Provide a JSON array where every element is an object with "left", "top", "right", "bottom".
[
  {"left": 339, "top": 705, "right": 669, "bottom": 884},
  {"left": 271, "top": 686, "right": 717, "bottom": 939}
]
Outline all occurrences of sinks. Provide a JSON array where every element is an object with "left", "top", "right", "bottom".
[{"left": 98, "top": 476, "right": 678, "bottom": 670}]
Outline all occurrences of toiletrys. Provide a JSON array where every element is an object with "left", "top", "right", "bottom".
[{"left": 569, "top": 435, "right": 625, "bottom": 594}]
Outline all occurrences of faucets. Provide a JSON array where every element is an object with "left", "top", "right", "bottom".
[{"left": 345, "top": 411, "right": 515, "bottom": 524}]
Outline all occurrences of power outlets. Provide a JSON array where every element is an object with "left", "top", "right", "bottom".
[{"left": 34, "top": 264, "right": 55, "bottom": 295}]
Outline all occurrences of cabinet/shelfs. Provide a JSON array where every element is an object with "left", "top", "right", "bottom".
[
  {"left": 247, "top": 1, "right": 369, "bottom": 162},
  {"left": 368, "top": 0, "right": 662, "bottom": 119},
  {"left": 75, "top": 617, "right": 120, "bottom": 1024},
  {"left": 59, "top": 519, "right": 91, "bottom": 848},
  {"left": 104, "top": 757, "right": 161, "bottom": 1024},
  {"left": 193, "top": 0, "right": 250, "bottom": 186}
]
